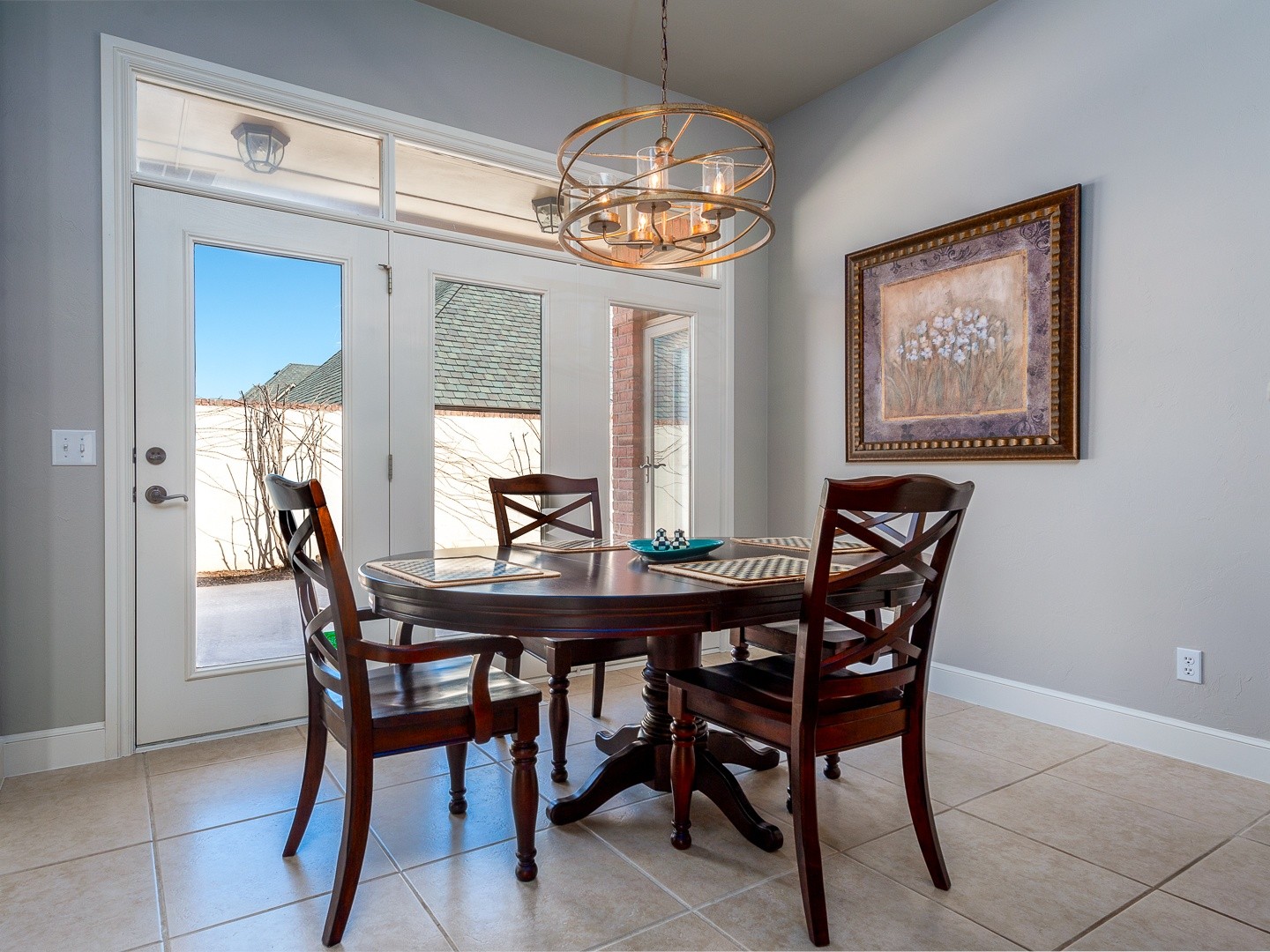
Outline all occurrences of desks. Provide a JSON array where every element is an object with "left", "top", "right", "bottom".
[{"left": 357, "top": 538, "right": 933, "bottom": 852}]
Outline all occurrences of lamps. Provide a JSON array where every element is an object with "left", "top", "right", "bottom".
[
  {"left": 557, "top": 3, "right": 778, "bottom": 272},
  {"left": 534, "top": 196, "right": 566, "bottom": 236},
  {"left": 232, "top": 122, "right": 289, "bottom": 174}
]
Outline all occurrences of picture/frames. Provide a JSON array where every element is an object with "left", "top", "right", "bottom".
[{"left": 845, "top": 181, "right": 1079, "bottom": 461}]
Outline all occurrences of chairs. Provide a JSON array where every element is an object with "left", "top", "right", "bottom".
[
  {"left": 488, "top": 473, "right": 644, "bottom": 784},
  {"left": 667, "top": 473, "right": 975, "bottom": 945},
  {"left": 260, "top": 473, "right": 542, "bottom": 945}
]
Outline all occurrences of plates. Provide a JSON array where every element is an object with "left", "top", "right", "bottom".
[{"left": 627, "top": 538, "right": 724, "bottom": 562}]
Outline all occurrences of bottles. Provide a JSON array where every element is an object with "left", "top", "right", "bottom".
[
  {"left": 671, "top": 529, "right": 690, "bottom": 549},
  {"left": 651, "top": 528, "right": 670, "bottom": 550}
]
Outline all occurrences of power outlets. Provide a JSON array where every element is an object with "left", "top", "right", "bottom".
[{"left": 1174, "top": 647, "right": 1202, "bottom": 684}]
